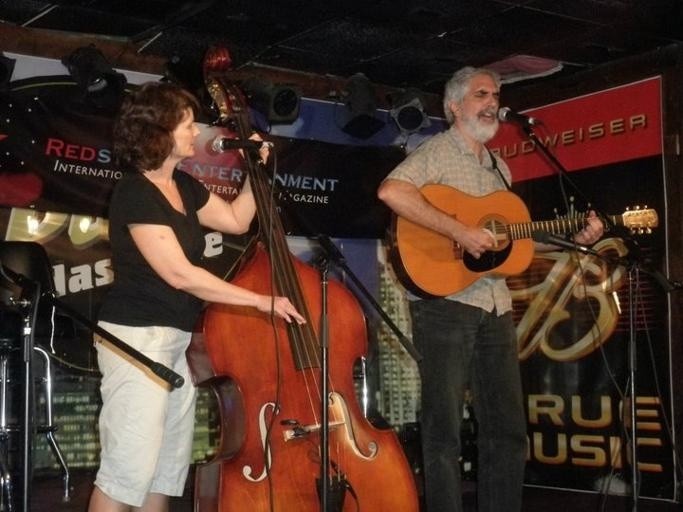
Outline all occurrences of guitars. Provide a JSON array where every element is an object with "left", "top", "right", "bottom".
[{"left": 385, "top": 183, "right": 658, "bottom": 300}]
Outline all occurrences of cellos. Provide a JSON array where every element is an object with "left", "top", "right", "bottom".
[{"left": 183, "top": 43, "right": 424, "bottom": 512}]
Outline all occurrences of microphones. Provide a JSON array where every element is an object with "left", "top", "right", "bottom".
[
  {"left": 532, "top": 228, "right": 596, "bottom": 255},
  {"left": 497, "top": 106, "right": 539, "bottom": 125},
  {"left": 210, "top": 134, "right": 275, "bottom": 154}
]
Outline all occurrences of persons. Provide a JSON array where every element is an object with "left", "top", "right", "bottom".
[
  {"left": 88, "top": 80, "right": 308, "bottom": 512},
  {"left": 376, "top": 66, "right": 606, "bottom": 512}
]
